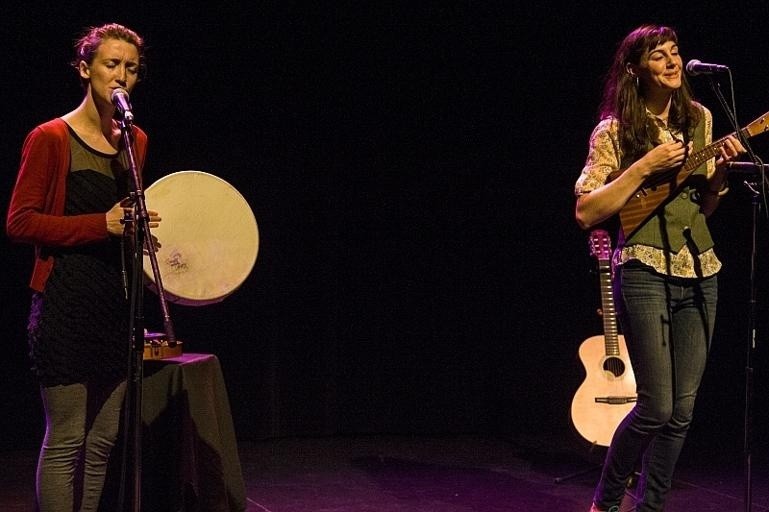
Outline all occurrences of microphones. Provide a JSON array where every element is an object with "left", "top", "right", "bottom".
[
  {"left": 109, "top": 85, "right": 134, "bottom": 121},
  {"left": 686, "top": 60, "right": 726, "bottom": 76}
]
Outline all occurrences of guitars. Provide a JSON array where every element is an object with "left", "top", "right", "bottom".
[
  {"left": 570, "top": 229, "right": 637, "bottom": 447},
  {"left": 608, "top": 112, "right": 769, "bottom": 239},
  {"left": 143, "top": 338, "right": 184, "bottom": 362}
]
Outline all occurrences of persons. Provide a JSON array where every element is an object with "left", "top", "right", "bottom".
[
  {"left": 570, "top": 24, "right": 750, "bottom": 511},
  {"left": 3, "top": 22, "right": 162, "bottom": 510}
]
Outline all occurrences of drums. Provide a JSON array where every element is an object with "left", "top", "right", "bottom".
[{"left": 132, "top": 169, "right": 261, "bottom": 305}]
